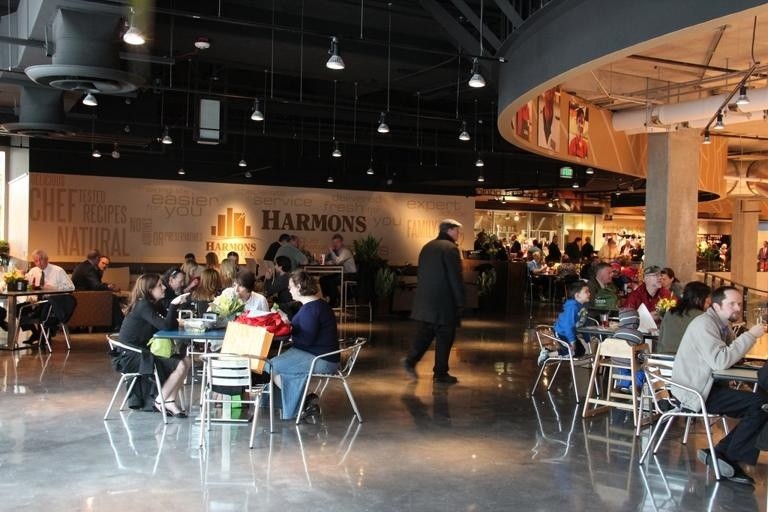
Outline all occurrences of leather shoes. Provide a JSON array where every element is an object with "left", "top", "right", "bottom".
[
  {"left": 725, "top": 460, "right": 758, "bottom": 486},
  {"left": 696, "top": 447, "right": 736, "bottom": 479}
]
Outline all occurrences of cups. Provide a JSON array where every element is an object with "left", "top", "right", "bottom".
[
  {"left": 599, "top": 313, "right": 609, "bottom": 329},
  {"left": 754, "top": 313, "right": 762, "bottom": 326}
]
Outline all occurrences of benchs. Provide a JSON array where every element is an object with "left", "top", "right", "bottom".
[{"left": 68, "top": 266, "right": 131, "bottom": 334}]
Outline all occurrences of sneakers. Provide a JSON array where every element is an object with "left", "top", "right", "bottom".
[
  {"left": 400, "top": 357, "right": 420, "bottom": 380},
  {"left": 294, "top": 392, "right": 320, "bottom": 422},
  {"left": 432, "top": 372, "right": 458, "bottom": 384},
  {"left": 213, "top": 394, "right": 250, "bottom": 409},
  {"left": 537, "top": 346, "right": 550, "bottom": 366},
  {"left": 22, "top": 332, "right": 52, "bottom": 346}
]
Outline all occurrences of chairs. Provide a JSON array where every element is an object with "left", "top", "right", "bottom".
[
  {"left": 13, "top": 296, "right": 77, "bottom": 352},
  {"left": 103, "top": 258, "right": 373, "bottom": 449},
  {"left": 523, "top": 254, "right": 768, "bottom": 481}
]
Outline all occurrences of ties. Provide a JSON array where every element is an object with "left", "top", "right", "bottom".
[{"left": 37, "top": 270, "right": 45, "bottom": 305}]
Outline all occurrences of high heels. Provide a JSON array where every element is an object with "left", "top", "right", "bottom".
[
  {"left": 152, "top": 398, "right": 173, "bottom": 417},
  {"left": 163, "top": 400, "right": 189, "bottom": 419}
]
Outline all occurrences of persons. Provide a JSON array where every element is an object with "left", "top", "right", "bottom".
[{"left": 402, "top": 219, "right": 466, "bottom": 385}]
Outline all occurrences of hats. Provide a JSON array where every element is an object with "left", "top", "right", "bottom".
[
  {"left": 660, "top": 267, "right": 681, "bottom": 284},
  {"left": 643, "top": 265, "right": 662, "bottom": 275}
]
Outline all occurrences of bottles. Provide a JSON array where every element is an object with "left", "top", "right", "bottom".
[{"left": 271, "top": 302, "right": 280, "bottom": 313}]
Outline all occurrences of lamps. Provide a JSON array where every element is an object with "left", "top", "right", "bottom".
[
  {"left": 332, "top": 149, "right": 342, "bottom": 157},
  {"left": 112, "top": 147, "right": 121, "bottom": 159},
  {"left": 122, "top": 12, "right": 148, "bottom": 46},
  {"left": 586, "top": 167, "right": 594, "bottom": 175},
  {"left": 162, "top": 132, "right": 172, "bottom": 145},
  {"left": 326, "top": 37, "right": 345, "bottom": 69},
  {"left": 377, "top": 117, "right": 390, "bottom": 133},
  {"left": 83, "top": 93, "right": 98, "bottom": 106},
  {"left": 92, "top": 150, "right": 102, "bottom": 158},
  {"left": 475, "top": 159, "right": 484, "bottom": 166},
  {"left": 194, "top": 36, "right": 210, "bottom": 50},
  {"left": 501, "top": 191, "right": 573, "bottom": 212},
  {"left": 239, "top": 161, "right": 247, "bottom": 167},
  {"left": 251, "top": 101, "right": 264, "bottom": 121},
  {"left": 573, "top": 180, "right": 579, "bottom": 188},
  {"left": 459, "top": 126, "right": 471, "bottom": 140},
  {"left": 468, "top": 66, "right": 485, "bottom": 88},
  {"left": 700, "top": 62, "right": 762, "bottom": 147}
]
